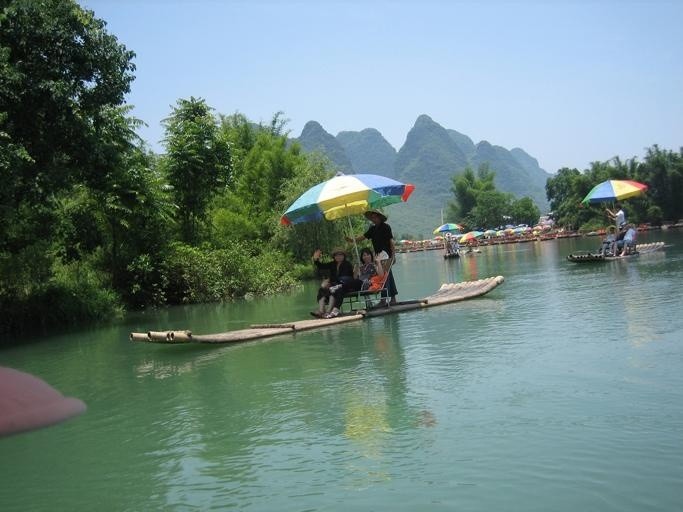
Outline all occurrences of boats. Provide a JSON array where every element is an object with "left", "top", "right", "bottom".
[
  {"left": 568, "top": 241, "right": 667, "bottom": 265},
  {"left": 442, "top": 248, "right": 482, "bottom": 258},
  {"left": 127, "top": 275, "right": 505, "bottom": 344}
]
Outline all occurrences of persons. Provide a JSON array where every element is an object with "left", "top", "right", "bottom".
[
  {"left": 601, "top": 203, "right": 638, "bottom": 256},
  {"left": 466, "top": 239, "right": 480, "bottom": 253},
  {"left": 309, "top": 246, "right": 354, "bottom": 319},
  {"left": 444, "top": 236, "right": 460, "bottom": 255},
  {"left": 322, "top": 247, "right": 384, "bottom": 318},
  {"left": 345, "top": 208, "right": 398, "bottom": 310}
]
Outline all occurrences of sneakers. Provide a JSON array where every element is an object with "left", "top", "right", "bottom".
[
  {"left": 329, "top": 286, "right": 337, "bottom": 293},
  {"left": 310, "top": 309, "right": 340, "bottom": 318}
]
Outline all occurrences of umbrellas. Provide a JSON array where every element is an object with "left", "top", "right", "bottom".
[
  {"left": 397, "top": 222, "right": 552, "bottom": 244},
  {"left": 578, "top": 179, "right": 650, "bottom": 226},
  {"left": 279, "top": 170, "right": 415, "bottom": 267}
]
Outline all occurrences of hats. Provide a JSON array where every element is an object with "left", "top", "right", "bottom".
[
  {"left": 365, "top": 209, "right": 387, "bottom": 222},
  {"left": 330, "top": 247, "right": 348, "bottom": 258},
  {"left": 606, "top": 224, "right": 616, "bottom": 233}
]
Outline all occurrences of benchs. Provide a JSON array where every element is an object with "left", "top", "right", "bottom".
[
  {"left": 599, "top": 232, "right": 637, "bottom": 254},
  {"left": 340, "top": 258, "right": 394, "bottom": 316}
]
